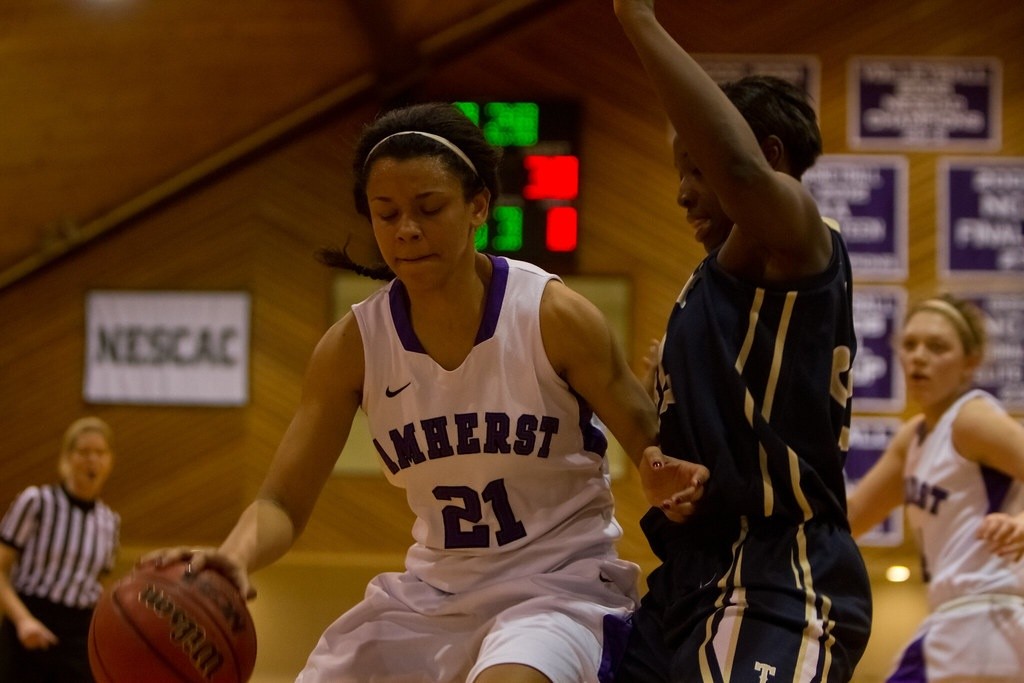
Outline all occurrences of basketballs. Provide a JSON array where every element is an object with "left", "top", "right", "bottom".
[{"left": 84, "top": 549, "right": 261, "bottom": 683}]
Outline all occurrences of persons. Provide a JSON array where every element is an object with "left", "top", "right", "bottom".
[
  {"left": 136, "top": 0, "right": 874, "bottom": 683},
  {"left": 848, "top": 294, "right": 1024, "bottom": 683},
  {"left": 0, "top": 416, "right": 122, "bottom": 683}
]
